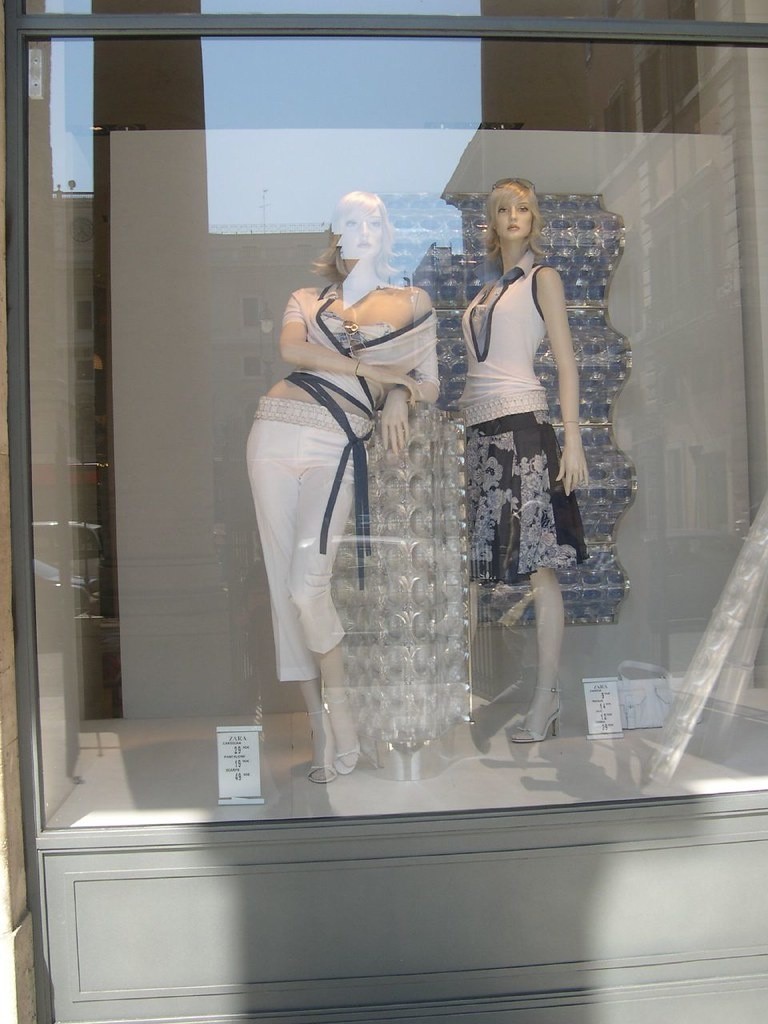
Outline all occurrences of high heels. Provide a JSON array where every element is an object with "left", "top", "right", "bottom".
[
  {"left": 323, "top": 688, "right": 361, "bottom": 775},
  {"left": 506, "top": 688, "right": 562, "bottom": 743},
  {"left": 305, "top": 709, "right": 339, "bottom": 783}
]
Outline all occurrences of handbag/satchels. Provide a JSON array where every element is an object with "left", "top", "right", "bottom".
[{"left": 616, "top": 659, "right": 686, "bottom": 729}]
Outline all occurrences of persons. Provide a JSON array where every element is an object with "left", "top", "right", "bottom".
[
  {"left": 450, "top": 178, "right": 589, "bottom": 744},
  {"left": 246, "top": 189, "right": 438, "bottom": 784}
]
